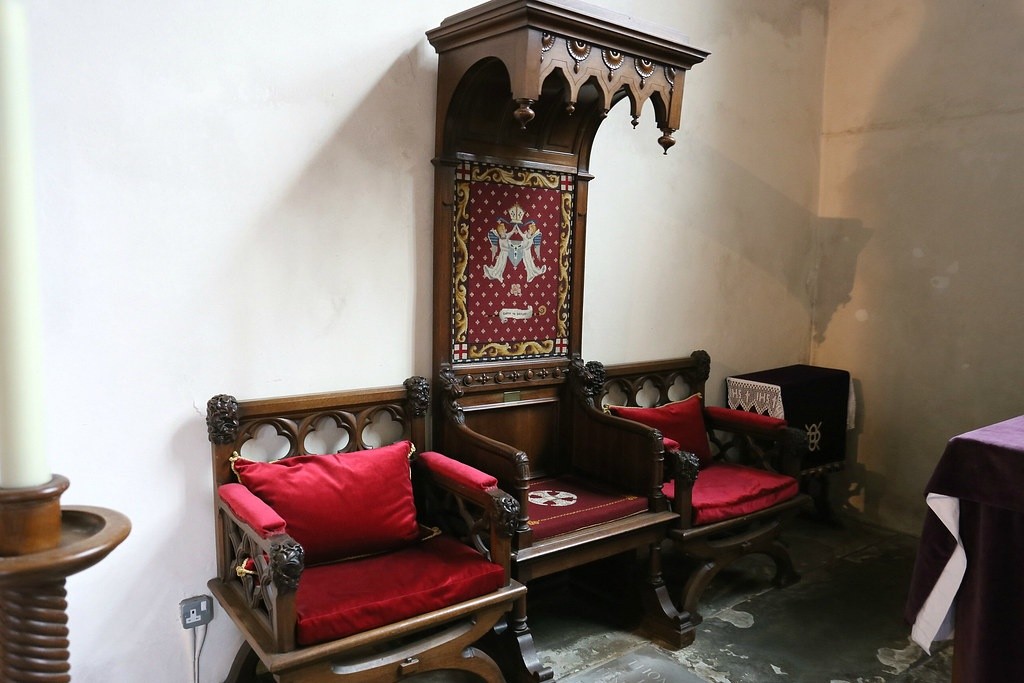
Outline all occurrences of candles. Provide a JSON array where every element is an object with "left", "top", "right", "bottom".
[{"left": 1, "top": 0, "right": 54, "bottom": 490}]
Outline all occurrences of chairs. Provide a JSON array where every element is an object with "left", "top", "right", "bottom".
[
  {"left": 583, "top": 344, "right": 808, "bottom": 626},
  {"left": 198, "top": 370, "right": 531, "bottom": 683},
  {"left": 433, "top": 361, "right": 697, "bottom": 683}
]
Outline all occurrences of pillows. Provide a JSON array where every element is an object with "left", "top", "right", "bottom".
[
  {"left": 603, "top": 391, "right": 714, "bottom": 483},
  {"left": 229, "top": 438, "right": 441, "bottom": 579}
]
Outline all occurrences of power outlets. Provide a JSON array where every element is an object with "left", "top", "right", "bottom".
[{"left": 180, "top": 595, "right": 213, "bottom": 629}]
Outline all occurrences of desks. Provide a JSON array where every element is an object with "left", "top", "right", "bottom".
[{"left": 722, "top": 358, "right": 859, "bottom": 524}]
